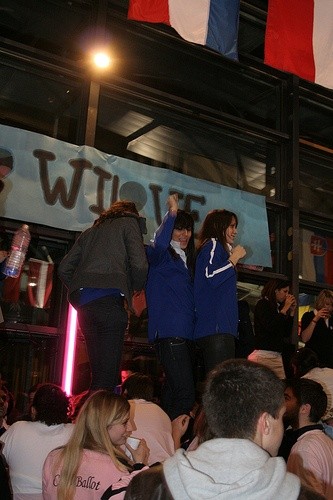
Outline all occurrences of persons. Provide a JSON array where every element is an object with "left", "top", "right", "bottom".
[
  {"left": 39, "top": 389, "right": 151, "bottom": 500},
  {"left": 145, "top": 193, "right": 198, "bottom": 451},
  {"left": 1, "top": 383, "right": 75, "bottom": 500},
  {"left": 193, "top": 208, "right": 248, "bottom": 417},
  {"left": 282, "top": 376, "right": 333, "bottom": 500},
  {"left": 118, "top": 371, "right": 175, "bottom": 467},
  {"left": 300, "top": 289, "right": 333, "bottom": 379},
  {"left": 122, "top": 358, "right": 330, "bottom": 500},
  {"left": 57, "top": 200, "right": 149, "bottom": 397},
  {"left": 253, "top": 277, "right": 299, "bottom": 388},
  {"left": 1, "top": 371, "right": 14, "bottom": 500}
]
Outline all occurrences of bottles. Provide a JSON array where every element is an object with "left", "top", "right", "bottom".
[{"left": 2, "top": 224, "right": 31, "bottom": 278}]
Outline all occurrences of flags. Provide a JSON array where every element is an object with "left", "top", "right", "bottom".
[
  {"left": 126, "top": 0, "right": 240, "bottom": 63},
  {"left": 264, "top": 0, "right": 332, "bottom": 90}
]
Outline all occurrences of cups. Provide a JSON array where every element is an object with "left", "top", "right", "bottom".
[
  {"left": 289, "top": 296, "right": 296, "bottom": 309},
  {"left": 125, "top": 437, "right": 141, "bottom": 457},
  {"left": 323, "top": 307, "right": 329, "bottom": 319}
]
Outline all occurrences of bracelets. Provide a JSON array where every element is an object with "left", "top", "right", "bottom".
[{"left": 312, "top": 319, "right": 317, "bottom": 324}]
